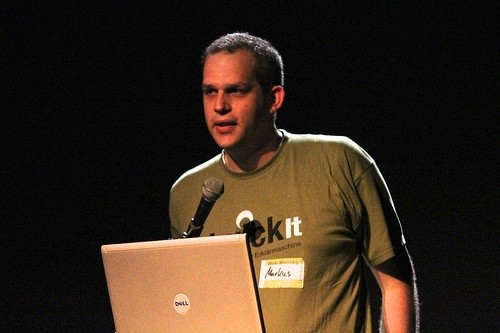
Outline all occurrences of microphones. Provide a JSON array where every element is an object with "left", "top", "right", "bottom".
[{"left": 182, "top": 177, "right": 224, "bottom": 238}]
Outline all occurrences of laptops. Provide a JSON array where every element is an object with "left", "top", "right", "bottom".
[{"left": 101, "top": 233, "right": 266, "bottom": 333}]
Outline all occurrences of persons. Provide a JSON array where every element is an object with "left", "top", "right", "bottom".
[{"left": 169, "top": 30, "right": 419, "bottom": 333}]
